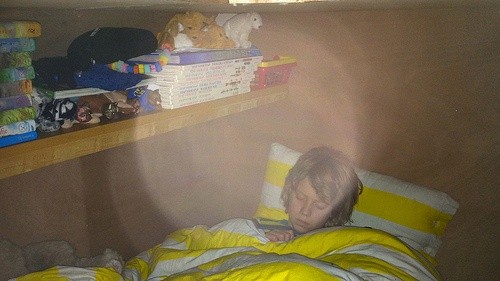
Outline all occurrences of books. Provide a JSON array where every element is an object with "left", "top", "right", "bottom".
[
  {"left": 127, "top": 48, "right": 264, "bottom": 110},
  {"left": 0, "top": 20, "right": 40, "bottom": 148}
]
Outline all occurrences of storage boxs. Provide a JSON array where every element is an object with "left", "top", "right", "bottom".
[{"left": 251, "top": 55, "right": 297, "bottom": 89}]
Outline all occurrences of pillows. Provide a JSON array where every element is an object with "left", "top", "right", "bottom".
[{"left": 242, "top": 142, "right": 459, "bottom": 260}]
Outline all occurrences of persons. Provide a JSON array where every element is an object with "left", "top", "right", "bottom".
[{"left": 251, "top": 145, "right": 365, "bottom": 242}]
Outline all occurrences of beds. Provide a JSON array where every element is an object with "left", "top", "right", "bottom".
[{"left": 7, "top": 142, "right": 459, "bottom": 281}]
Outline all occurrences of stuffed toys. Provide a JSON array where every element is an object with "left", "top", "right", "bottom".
[
  {"left": 162, "top": 10, "right": 236, "bottom": 53},
  {"left": 224, "top": 11, "right": 262, "bottom": 49}
]
